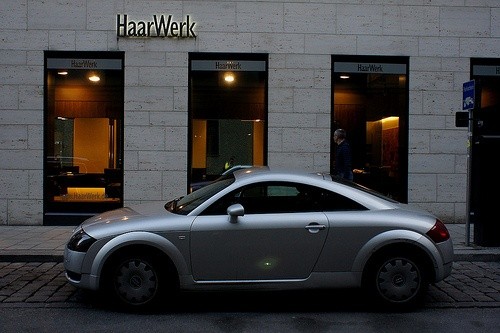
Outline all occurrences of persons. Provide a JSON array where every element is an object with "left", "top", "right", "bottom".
[{"left": 334, "top": 129, "right": 356, "bottom": 183}]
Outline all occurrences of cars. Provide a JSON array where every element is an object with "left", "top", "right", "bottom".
[{"left": 63, "top": 166, "right": 454, "bottom": 309}]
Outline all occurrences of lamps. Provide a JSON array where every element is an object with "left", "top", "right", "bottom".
[
  {"left": 224, "top": 72, "right": 234, "bottom": 82},
  {"left": 88, "top": 71, "right": 101, "bottom": 82}
]
